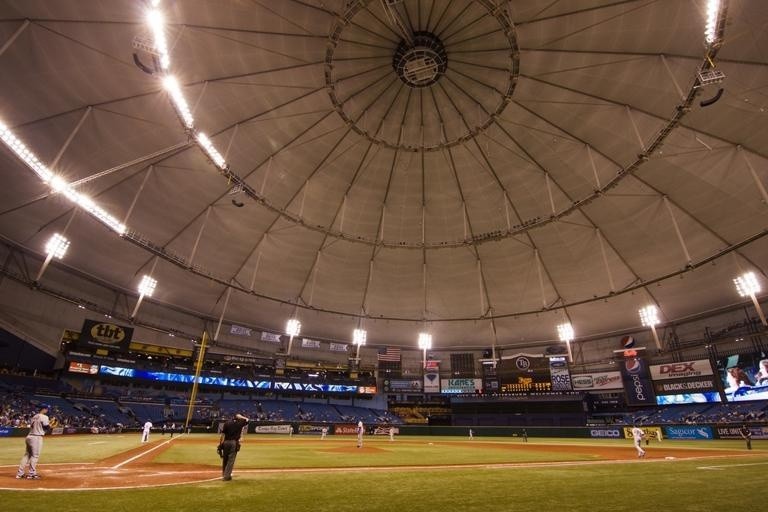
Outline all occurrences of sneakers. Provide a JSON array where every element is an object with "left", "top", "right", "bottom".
[
  {"left": 222, "top": 473, "right": 232, "bottom": 481},
  {"left": 638, "top": 451, "right": 646, "bottom": 458},
  {"left": 16, "top": 473, "right": 42, "bottom": 480}
]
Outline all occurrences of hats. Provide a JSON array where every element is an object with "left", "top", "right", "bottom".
[
  {"left": 146, "top": 418, "right": 152, "bottom": 421},
  {"left": 38, "top": 403, "right": 53, "bottom": 411},
  {"left": 633, "top": 423, "right": 639, "bottom": 428},
  {"left": 232, "top": 413, "right": 245, "bottom": 420}
]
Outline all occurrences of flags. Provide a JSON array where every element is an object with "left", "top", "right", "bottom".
[{"left": 377, "top": 346, "right": 400, "bottom": 361}]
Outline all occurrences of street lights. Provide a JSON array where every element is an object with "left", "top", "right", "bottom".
[
  {"left": 556, "top": 321, "right": 576, "bottom": 363},
  {"left": 732, "top": 271, "right": 768, "bottom": 327},
  {"left": 419, "top": 333, "right": 432, "bottom": 367},
  {"left": 638, "top": 304, "right": 662, "bottom": 352},
  {"left": 34, "top": 233, "right": 72, "bottom": 285},
  {"left": 283, "top": 319, "right": 302, "bottom": 356},
  {"left": 351, "top": 328, "right": 368, "bottom": 364},
  {"left": 129, "top": 274, "right": 159, "bottom": 319}
]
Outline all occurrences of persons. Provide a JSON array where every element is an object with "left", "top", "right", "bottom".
[
  {"left": 632, "top": 423, "right": 645, "bottom": 458},
  {"left": 475, "top": 384, "right": 504, "bottom": 397},
  {"left": 740, "top": 424, "right": 752, "bottom": 449},
  {"left": 655, "top": 429, "right": 662, "bottom": 442},
  {"left": 16, "top": 403, "right": 54, "bottom": 480},
  {"left": 356, "top": 419, "right": 363, "bottom": 449},
  {"left": 522, "top": 428, "right": 528, "bottom": 442},
  {"left": 723, "top": 358, "right": 768, "bottom": 401},
  {"left": 218, "top": 414, "right": 252, "bottom": 480},
  {"left": 468, "top": 428, "right": 475, "bottom": 440},
  {"left": 631, "top": 404, "right": 768, "bottom": 426}
]
